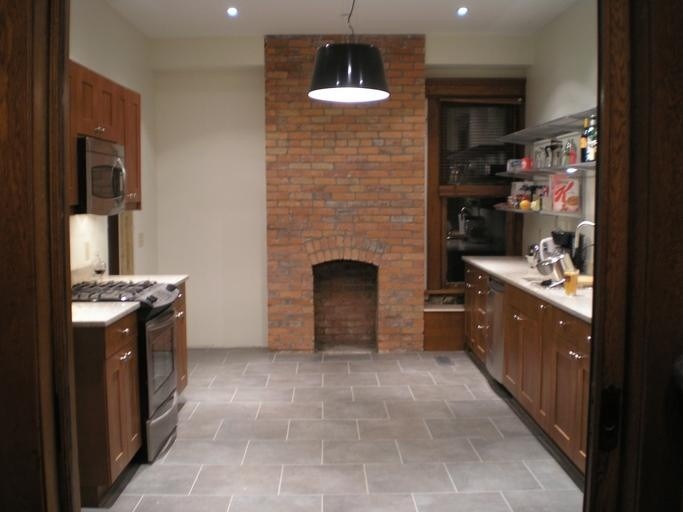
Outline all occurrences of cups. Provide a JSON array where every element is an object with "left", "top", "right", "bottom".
[
  {"left": 564, "top": 269, "right": 579, "bottom": 295},
  {"left": 448, "top": 156, "right": 501, "bottom": 184},
  {"left": 503, "top": 145, "right": 546, "bottom": 172}
]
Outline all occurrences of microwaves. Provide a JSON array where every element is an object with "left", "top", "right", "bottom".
[{"left": 82, "top": 136, "right": 130, "bottom": 216}]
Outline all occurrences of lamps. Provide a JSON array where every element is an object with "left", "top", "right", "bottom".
[{"left": 306, "top": 1, "right": 392, "bottom": 104}]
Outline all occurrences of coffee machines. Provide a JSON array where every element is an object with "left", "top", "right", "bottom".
[{"left": 551, "top": 230, "right": 583, "bottom": 273}]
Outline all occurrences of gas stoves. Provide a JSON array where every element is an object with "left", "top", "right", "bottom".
[{"left": 70, "top": 281, "right": 152, "bottom": 300}]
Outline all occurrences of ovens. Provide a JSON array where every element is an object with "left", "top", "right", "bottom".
[{"left": 138, "top": 282, "right": 181, "bottom": 461}]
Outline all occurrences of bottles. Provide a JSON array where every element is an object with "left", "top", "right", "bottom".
[{"left": 548, "top": 113, "right": 597, "bottom": 166}]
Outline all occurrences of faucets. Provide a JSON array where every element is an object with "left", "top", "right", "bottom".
[{"left": 575, "top": 220, "right": 596, "bottom": 248}]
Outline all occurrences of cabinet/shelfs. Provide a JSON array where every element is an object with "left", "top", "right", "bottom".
[
  {"left": 73, "top": 281, "right": 187, "bottom": 505},
  {"left": 426, "top": 76, "right": 526, "bottom": 296},
  {"left": 462, "top": 260, "right": 592, "bottom": 494},
  {"left": 494, "top": 106, "right": 596, "bottom": 225},
  {"left": 66, "top": 57, "right": 143, "bottom": 214}
]
